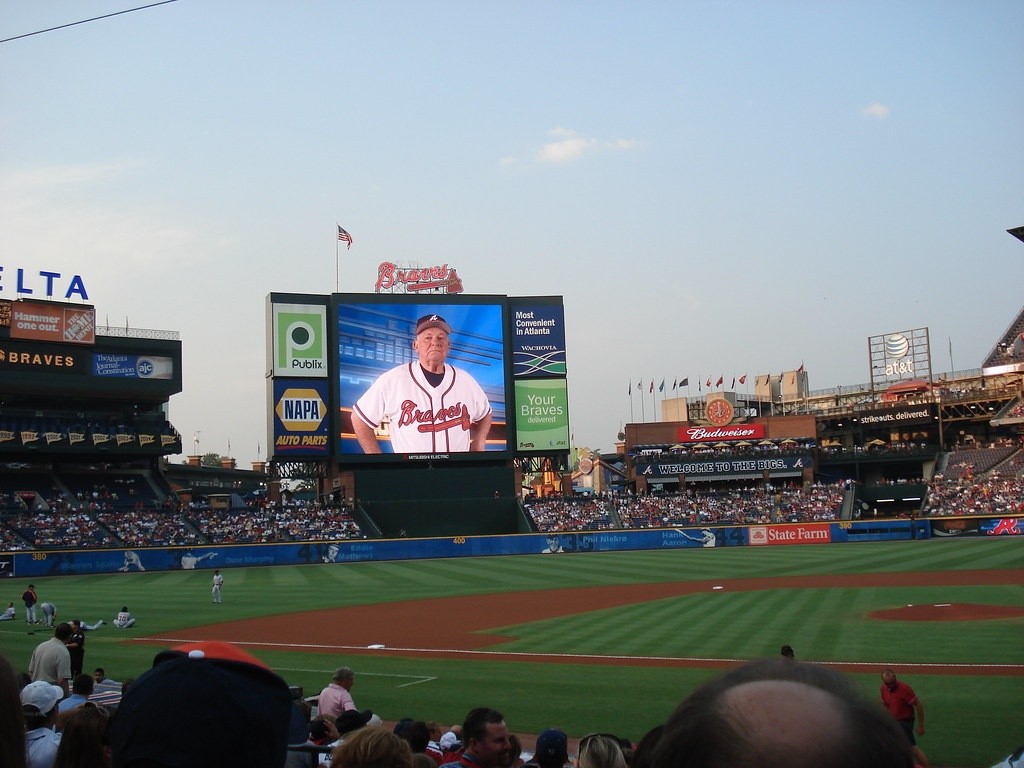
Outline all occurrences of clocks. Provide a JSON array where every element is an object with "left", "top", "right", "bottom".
[{"left": 706, "top": 397, "right": 734, "bottom": 426}]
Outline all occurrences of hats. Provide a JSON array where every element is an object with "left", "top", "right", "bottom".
[
  {"left": 536, "top": 729, "right": 567, "bottom": 757},
  {"left": 415, "top": 315, "right": 451, "bottom": 334},
  {"left": 366, "top": 714, "right": 383, "bottom": 727},
  {"left": 29, "top": 585, "right": 36, "bottom": 588},
  {"left": 19, "top": 681, "right": 64, "bottom": 716},
  {"left": 335, "top": 709, "right": 372, "bottom": 734}
]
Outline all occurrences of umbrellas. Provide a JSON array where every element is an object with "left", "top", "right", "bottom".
[
  {"left": 758, "top": 441, "right": 774, "bottom": 445},
  {"left": 826, "top": 440, "right": 842, "bottom": 450},
  {"left": 669, "top": 444, "right": 687, "bottom": 449},
  {"left": 781, "top": 439, "right": 795, "bottom": 443},
  {"left": 870, "top": 439, "right": 886, "bottom": 444},
  {"left": 735, "top": 441, "right": 752, "bottom": 446},
  {"left": 713, "top": 443, "right": 731, "bottom": 448},
  {"left": 692, "top": 443, "right": 709, "bottom": 448}
]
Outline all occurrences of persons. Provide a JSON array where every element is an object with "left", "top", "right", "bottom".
[
  {"left": 120, "top": 551, "right": 146, "bottom": 573},
  {"left": 541, "top": 536, "right": 564, "bottom": 553},
  {"left": 351, "top": 314, "right": 492, "bottom": 454},
  {"left": 181, "top": 550, "right": 212, "bottom": 569},
  {"left": 690, "top": 528, "right": 715, "bottom": 547},
  {"left": 1, "top": 482, "right": 362, "bottom": 550},
  {"left": 0, "top": 622, "right": 1024, "bottom": 768},
  {"left": 320, "top": 542, "right": 341, "bottom": 563},
  {"left": 513, "top": 439, "right": 922, "bottom": 531},
  {"left": 0, "top": 583, "right": 135, "bottom": 631},
  {"left": 922, "top": 398, "right": 1024, "bottom": 515},
  {"left": 212, "top": 570, "right": 223, "bottom": 603}
]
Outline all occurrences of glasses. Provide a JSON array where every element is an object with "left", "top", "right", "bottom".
[{"left": 578, "top": 733, "right": 621, "bottom": 757}]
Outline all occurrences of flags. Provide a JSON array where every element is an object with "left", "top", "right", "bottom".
[
  {"left": 628, "top": 366, "right": 803, "bottom": 395},
  {"left": 338, "top": 227, "right": 353, "bottom": 248}
]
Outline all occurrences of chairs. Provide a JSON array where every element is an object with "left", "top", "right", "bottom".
[
  {"left": 933, "top": 448, "right": 1024, "bottom": 478},
  {"left": 189, "top": 513, "right": 363, "bottom": 544},
  {"left": 0, "top": 474, "right": 164, "bottom": 550},
  {"left": 543, "top": 494, "right": 841, "bottom": 531}
]
